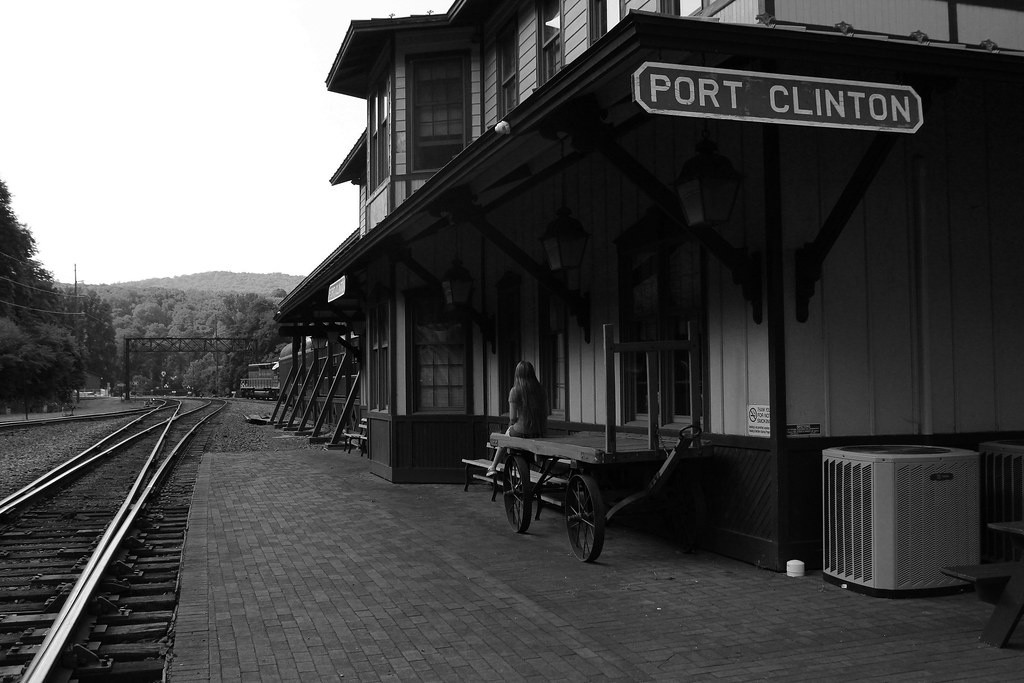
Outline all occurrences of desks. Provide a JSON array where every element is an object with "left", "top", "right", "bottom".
[{"left": 977, "top": 521, "right": 1024, "bottom": 646}]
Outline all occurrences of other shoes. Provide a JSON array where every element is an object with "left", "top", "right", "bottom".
[{"left": 485, "top": 468, "right": 497, "bottom": 476}]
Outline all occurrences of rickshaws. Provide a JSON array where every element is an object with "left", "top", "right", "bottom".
[{"left": 463, "top": 423, "right": 720, "bottom": 565}]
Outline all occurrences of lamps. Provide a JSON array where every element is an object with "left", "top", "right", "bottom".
[
  {"left": 310, "top": 299, "right": 327, "bottom": 350},
  {"left": 539, "top": 117, "right": 588, "bottom": 273},
  {"left": 438, "top": 208, "right": 475, "bottom": 304},
  {"left": 677, "top": 58, "right": 741, "bottom": 224},
  {"left": 351, "top": 283, "right": 371, "bottom": 337},
  {"left": 328, "top": 294, "right": 343, "bottom": 344}
]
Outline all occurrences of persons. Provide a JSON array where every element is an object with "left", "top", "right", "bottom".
[{"left": 485, "top": 360, "right": 547, "bottom": 477}]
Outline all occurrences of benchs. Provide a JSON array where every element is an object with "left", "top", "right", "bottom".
[
  {"left": 343, "top": 417, "right": 368, "bottom": 457},
  {"left": 456, "top": 442, "right": 573, "bottom": 520},
  {"left": 940, "top": 560, "right": 1017, "bottom": 584}
]
